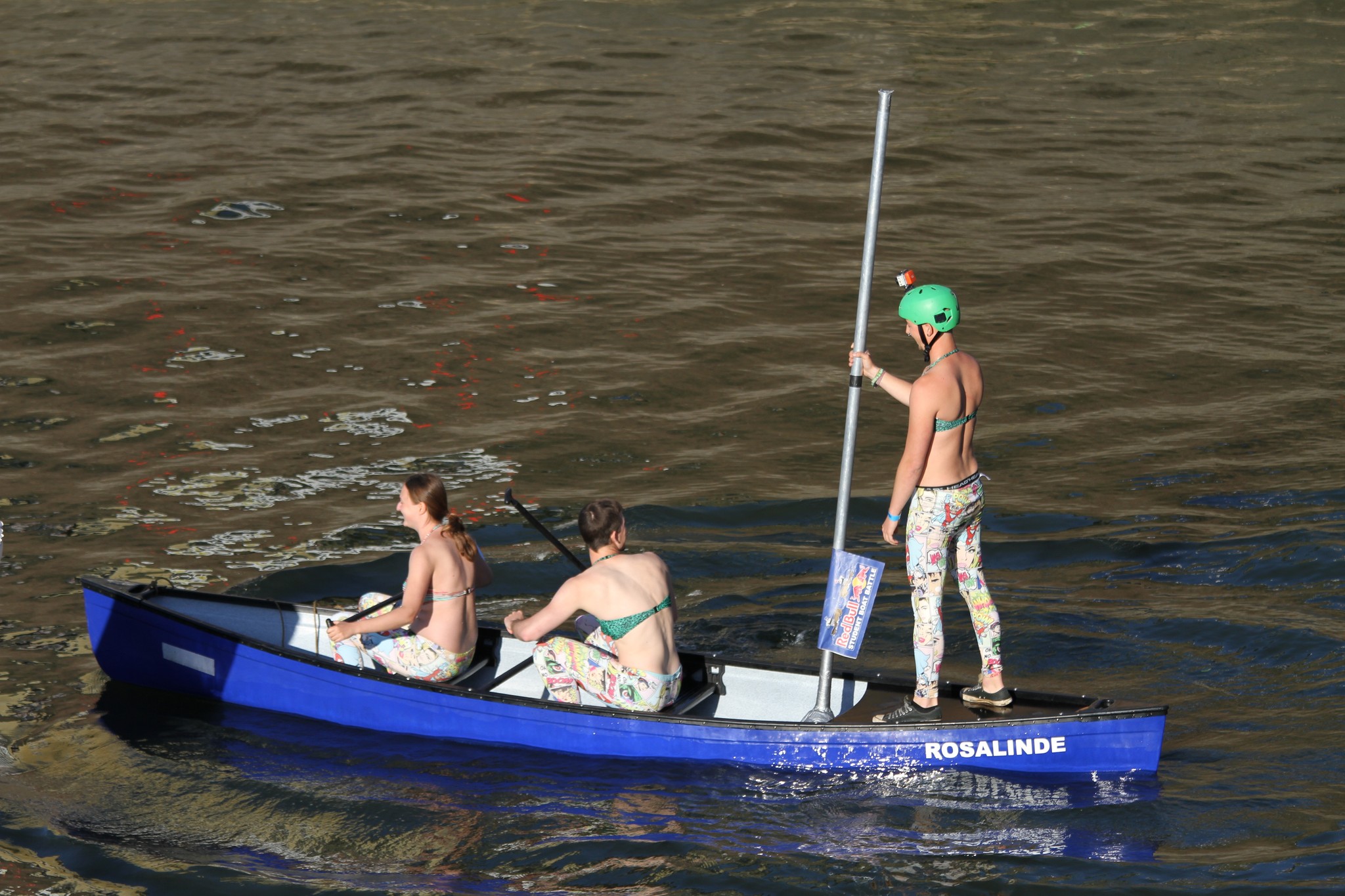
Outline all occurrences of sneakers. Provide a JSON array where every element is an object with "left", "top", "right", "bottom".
[
  {"left": 958, "top": 683, "right": 1013, "bottom": 707},
  {"left": 872, "top": 694, "right": 943, "bottom": 724}
]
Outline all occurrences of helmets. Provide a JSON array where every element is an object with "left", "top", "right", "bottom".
[{"left": 898, "top": 284, "right": 961, "bottom": 332}]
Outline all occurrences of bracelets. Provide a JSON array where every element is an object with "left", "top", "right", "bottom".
[
  {"left": 887, "top": 509, "right": 902, "bottom": 522},
  {"left": 868, "top": 366, "right": 885, "bottom": 388}
]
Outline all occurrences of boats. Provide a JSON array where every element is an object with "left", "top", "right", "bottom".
[{"left": 81, "top": 574, "right": 1169, "bottom": 779}]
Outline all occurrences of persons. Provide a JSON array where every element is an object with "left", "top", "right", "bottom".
[
  {"left": 848, "top": 283, "right": 1015, "bottom": 726},
  {"left": 503, "top": 498, "right": 684, "bottom": 713},
  {"left": 326, "top": 472, "right": 486, "bottom": 683}
]
